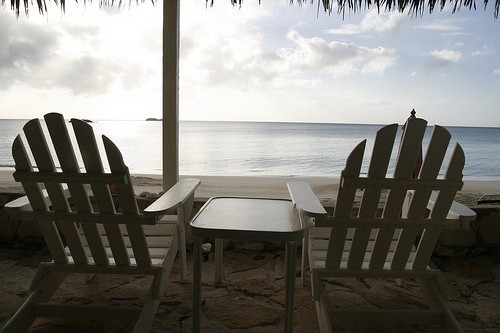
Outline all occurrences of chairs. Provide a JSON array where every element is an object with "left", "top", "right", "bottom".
[
  {"left": 287, "top": 116, "right": 478, "bottom": 332},
  {"left": 1, "top": 111, "right": 202, "bottom": 332}
]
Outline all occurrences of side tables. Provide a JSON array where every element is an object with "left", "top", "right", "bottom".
[{"left": 186, "top": 195, "right": 305, "bottom": 333}]
